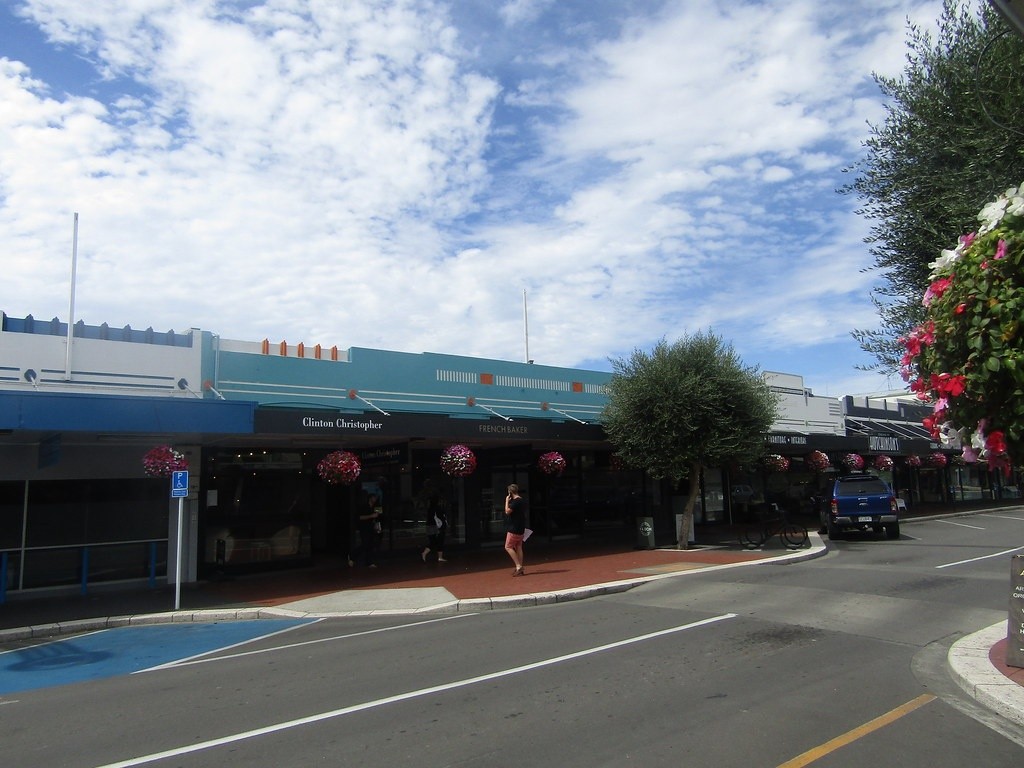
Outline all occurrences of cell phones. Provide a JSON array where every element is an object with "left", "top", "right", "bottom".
[{"left": 509, "top": 491, "right": 512, "bottom": 498}]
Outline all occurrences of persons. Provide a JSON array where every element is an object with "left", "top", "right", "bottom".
[
  {"left": 1015, "top": 478, "right": 1024, "bottom": 496},
  {"left": 348, "top": 493, "right": 380, "bottom": 568},
  {"left": 744, "top": 490, "right": 778, "bottom": 541},
  {"left": 421, "top": 496, "right": 447, "bottom": 562},
  {"left": 947, "top": 481, "right": 957, "bottom": 505},
  {"left": 991, "top": 482, "right": 1012, "bottom": 499},
  {"left": 505, "top": 484, "right": 527, "bottom": 576},
  {"left": 288, "top": 487, "right": 306, "bottom": 554}
]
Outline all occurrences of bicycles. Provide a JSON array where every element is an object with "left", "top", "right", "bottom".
[{"left": 737, "top": 508, "right": 809, "bottom": 550}]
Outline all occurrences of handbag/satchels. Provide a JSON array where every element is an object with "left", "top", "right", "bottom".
[
  {"left": 373, "top": 519, "right": 383, "bottom": 534},
  {"left": 433, "top": 505, "right": 449, "bottom": 529}
]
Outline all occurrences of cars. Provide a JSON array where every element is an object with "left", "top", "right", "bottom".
[{"left": 816, "top": 471, "right": 900, "bottom": 540}]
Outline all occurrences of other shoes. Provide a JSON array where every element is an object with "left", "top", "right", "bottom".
[
  {"left": 368, "top": 564, "right": 377, "bottom": 569},
  {"left": 420, "top": 553, "right": 426, "bottom": 563},
  {"left": 512, "top": 565, "right": 523, "bottom": 577},
  {"left": 438, "top": 558, "right": 447, "bottom": 562},
  {"left": 348, "top": 553, "right": 354, "bottom": 567}
]
